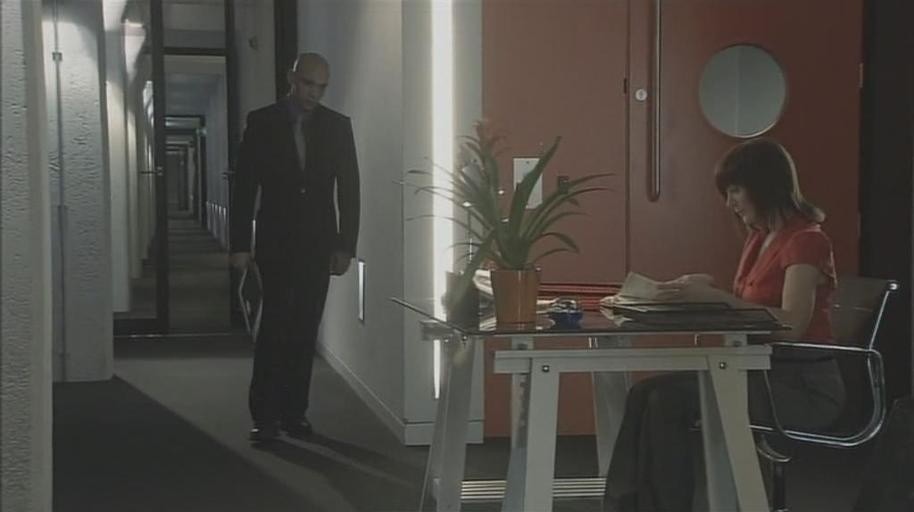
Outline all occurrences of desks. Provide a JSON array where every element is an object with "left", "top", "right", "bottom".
[{"left": 391, "top": 295, "right": 791, "bottom": 512}]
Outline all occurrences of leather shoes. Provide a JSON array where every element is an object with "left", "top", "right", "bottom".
[
  {"left": 247, "top": 419, "right": 276, "bottom": 440},
  {"left": 281, "top": 413, "right": 312, "bottom": 435}
]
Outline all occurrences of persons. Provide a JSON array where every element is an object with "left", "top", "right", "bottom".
[
  {"left": 605, "top": 136, "right": 837, "bottom": 511},
  {"left": 229, "top": 55, "right": 360, "bottom": 441}
]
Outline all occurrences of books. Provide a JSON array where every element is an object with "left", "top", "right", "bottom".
[{"left": 600, "top": 272, "right": 731, "bottom": 312}]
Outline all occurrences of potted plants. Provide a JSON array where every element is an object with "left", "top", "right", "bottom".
[{"left": 408, "top": 120, "right": 613, "bottom": 331}]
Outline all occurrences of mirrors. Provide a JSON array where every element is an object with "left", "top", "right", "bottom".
[{"left": 698, "top": 43, "right": 786, "bottom": 140}]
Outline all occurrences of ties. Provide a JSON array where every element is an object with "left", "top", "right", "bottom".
[{"left": 292, "top": 113, "right": 306, "bottom": 172}]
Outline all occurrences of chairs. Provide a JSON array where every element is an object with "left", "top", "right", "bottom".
[{"left": 690, "top": 276, "right": 902, "bottom": 512}]
